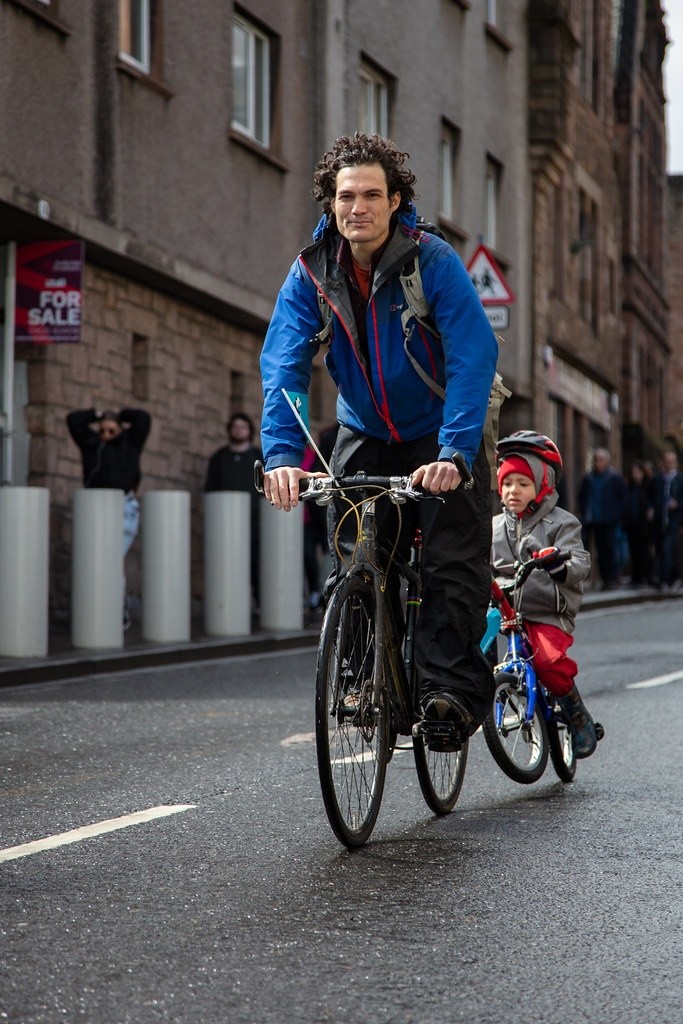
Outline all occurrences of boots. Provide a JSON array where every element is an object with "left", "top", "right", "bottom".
[{"left": 555, "top": 685, "right": 597, "bottom": 759}]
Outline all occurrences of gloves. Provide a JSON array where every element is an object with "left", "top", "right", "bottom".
[{"left": 533, "top": 545, "right": 566, "bottom": 578}]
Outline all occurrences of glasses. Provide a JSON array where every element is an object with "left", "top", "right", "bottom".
[{"left": 98, "top": 427, "right": 120, "bottom": 434}]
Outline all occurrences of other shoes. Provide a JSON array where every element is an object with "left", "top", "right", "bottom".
[
  {"left": 422, "top": 695, "right": 463, "bottom": 754},
  {"left": 344, "top": 694, "right": 364, "bottom": 723}
]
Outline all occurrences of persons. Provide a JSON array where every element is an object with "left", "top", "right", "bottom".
[
  {"left": 582, "top": 448, "right": 683, "bottom": 590},
  {"left": 260, "top": 133, "right": 499, "bottom": 751},
  {"left": 66, "top": 407, "right": 151, "bottom": 630},
  {"left": 492, "top": 430, "right": 597, "bottom": 758},
  {"left": 203, "top": 412, "right": 263, "bottom": 492}
]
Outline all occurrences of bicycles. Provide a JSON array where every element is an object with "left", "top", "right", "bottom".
[
  {"left": 478, "top": 549, "right": 606, "bottom": 783},
  {"left": 251, "top": 451, "right": 478, "bottom": 851}
]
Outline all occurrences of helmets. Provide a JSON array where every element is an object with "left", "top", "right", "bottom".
[{"left": 493, "top": 429, "right": 564, "bottom": 492}]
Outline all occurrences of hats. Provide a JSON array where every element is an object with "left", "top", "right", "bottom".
[{"left": 497, "top": 456, "right": 533, "bottom": 495}]
